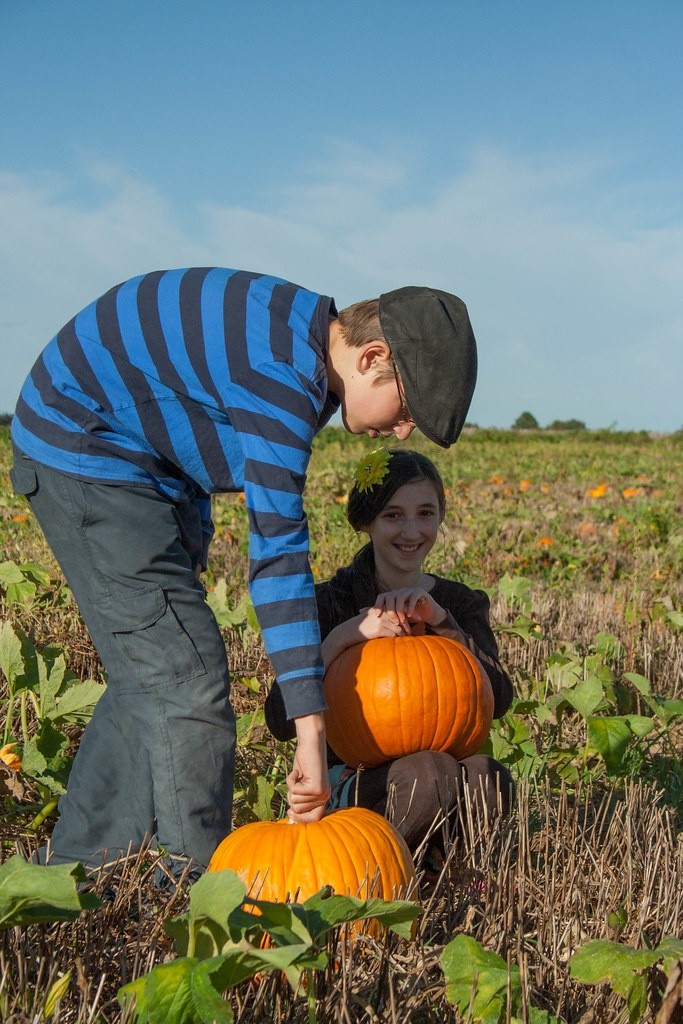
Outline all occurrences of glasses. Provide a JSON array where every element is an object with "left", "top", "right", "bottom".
[{"left": 391, "top": 356, "right": 414, "bottom": 423}]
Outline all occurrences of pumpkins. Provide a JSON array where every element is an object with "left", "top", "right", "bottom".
[
  {"left": 203, "top": 806, "right": 418, "bottom": 987},
  {"left": 322, "top": 597, "right": 495, "bottom": 769}
]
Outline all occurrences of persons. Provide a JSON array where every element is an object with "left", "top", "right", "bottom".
[
  {"left": 265, "top": 450, "right": 514, "bottom": 883},
  {"left": 11, "top": 268, "right": 477, "bottom": 903}
]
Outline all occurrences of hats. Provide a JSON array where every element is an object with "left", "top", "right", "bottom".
[{"left": 378, "top": 285, "right": 479, "bottom": 451}]
